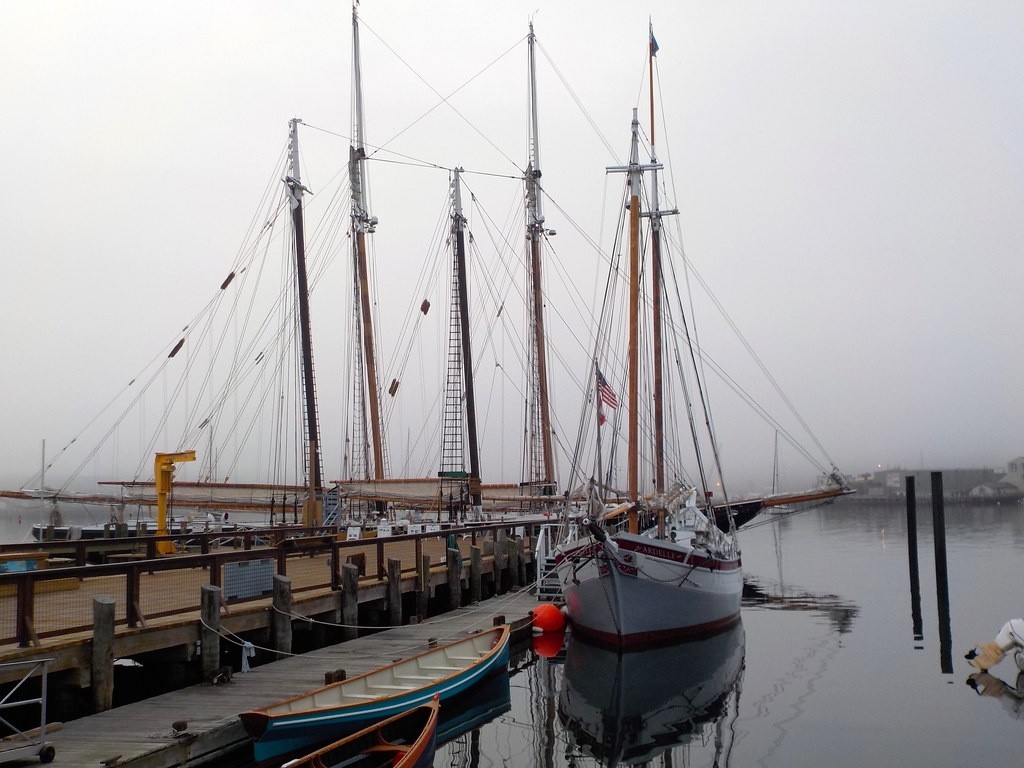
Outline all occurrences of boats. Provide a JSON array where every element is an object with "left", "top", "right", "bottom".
[
  {"left": 280, "top": 692, "right": 442, "bottom": 768},
  {"left": 95, "top": 3, "right": 375, "bottom": 508},
  {"left": 0, "top": 485, "right": 133, "bottom": 509},
  {"left": 238, "top": 621, "right": 513, "bottom": 743},
  {"left": 551, "top": 106, "right": 744, "bottom": 655},
  {"left": 331, "top": 469, "right": 532, "bottom": 510},
  {"left": 29, "top": 115, "right": 338, "bottom": 544},
  {"left": 644, "top": 22, "right": 858, "bottom": 541}
]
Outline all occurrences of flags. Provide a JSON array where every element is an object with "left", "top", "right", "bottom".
[{"left": 598, "top": 369, "right": 618, "bottom": 409}]
